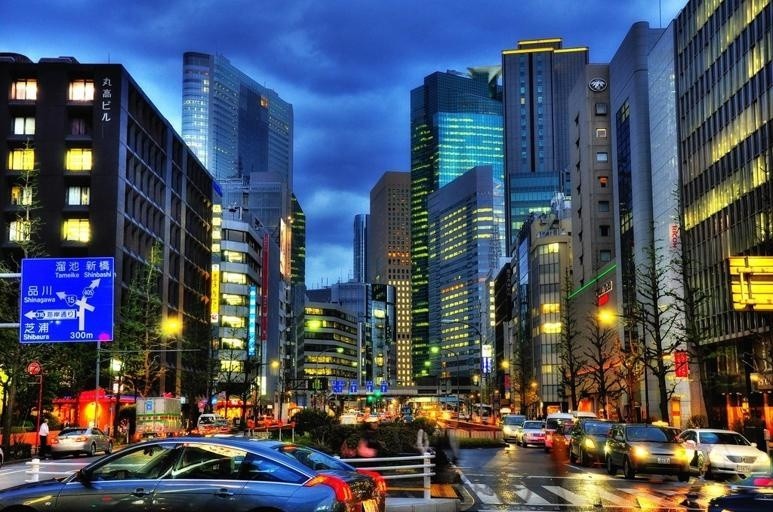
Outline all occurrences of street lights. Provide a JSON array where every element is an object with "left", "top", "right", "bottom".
[
  {"left": 438, "top": 318, "right": 482, "bottom": 424},
  {"left": 314, "top": 345, "right": 367, "bottom": 393},
  {"left": 253, "top": 358, "right": 281, "bottom": 422},
  {"left": 411, "top": 344, "right": 459, "bottom": 423}
]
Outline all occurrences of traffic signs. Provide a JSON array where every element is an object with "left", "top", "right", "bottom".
[
  {"left": 20, "top": 257, "right": 114, "bottom": 342},
  {"left": 366, "top": 381, "right": 373, "bottom": 393},
  {"left": 380, "top": 380, "right": 387, "bottom": 392}
]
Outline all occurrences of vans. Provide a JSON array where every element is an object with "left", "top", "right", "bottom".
[{"left": 0, "top": 419, "right": 35, "bottom": 433}]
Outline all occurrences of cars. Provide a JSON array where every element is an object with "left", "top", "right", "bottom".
[
  {"left": 705, "top": 473, "right": 773, "bottom": 512},
  {"left": 49, "top": 427, "right": 116, "bottom": 458},
  {"left": 192, "top": 408, "right": 415, "bottom": 436},
  {"left": 0, "top": 446, "right": 4, "bottom": 469},
  {"left": 136, "top": 434, "right": 390, "bottom": 512},
  {"left": 0, "top": 432, "right": 352, "bottom": 512}
]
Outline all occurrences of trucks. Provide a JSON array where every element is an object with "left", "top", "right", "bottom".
[{"left": 133, "top": 395, "right": 186, "bottom": 444}]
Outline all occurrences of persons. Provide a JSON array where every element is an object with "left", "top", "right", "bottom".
[{"left": 38, "top": 419, "right": 50, "bottom": 457}]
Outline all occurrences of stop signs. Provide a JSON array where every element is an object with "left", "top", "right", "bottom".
[{"left": 28, "top": 362, "right": 40, "bottom": 377}]
